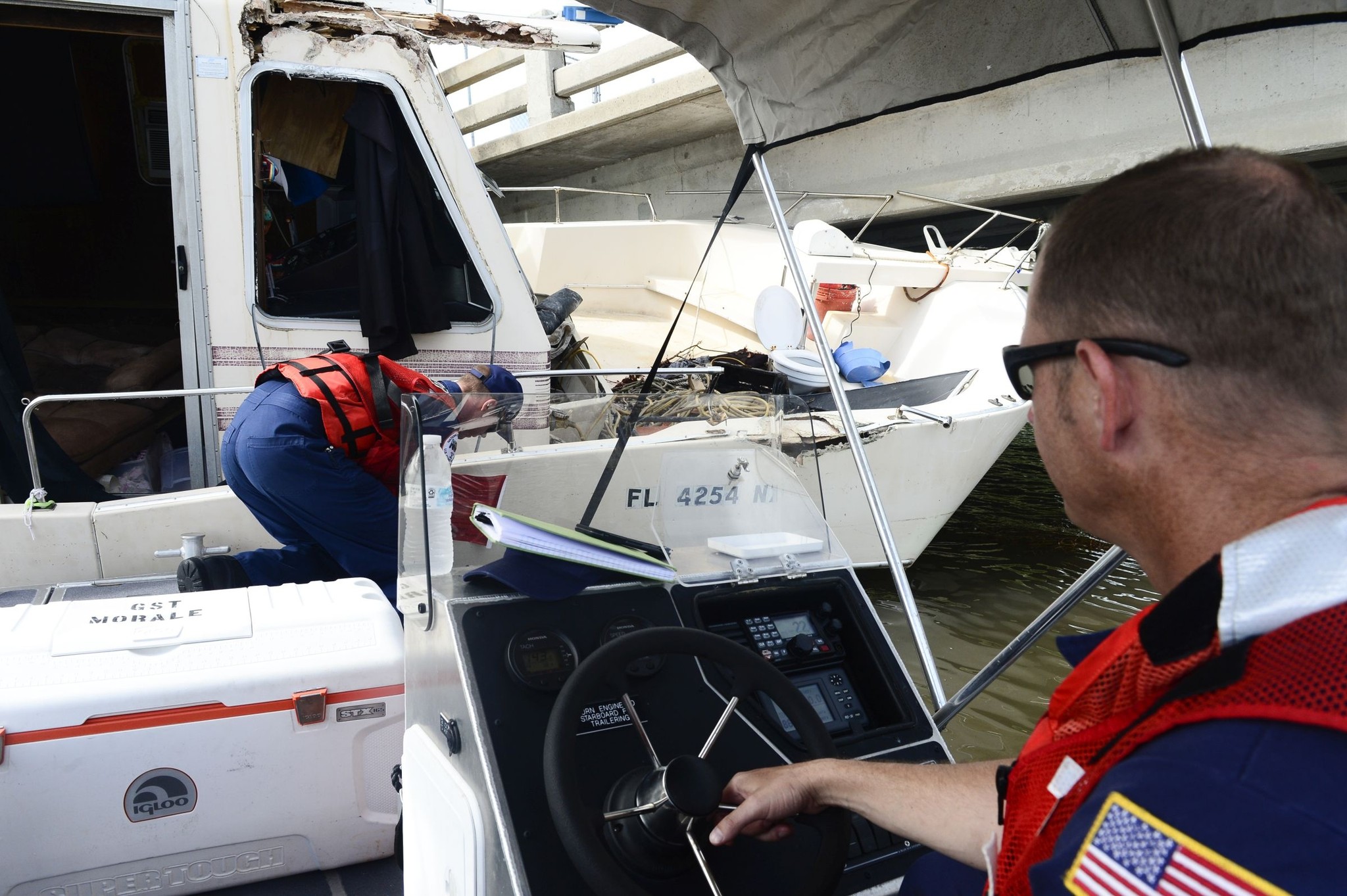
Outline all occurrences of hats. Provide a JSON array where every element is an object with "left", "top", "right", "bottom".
[{"left": 471, "top": 365, "right": 523, "bottom": 448}]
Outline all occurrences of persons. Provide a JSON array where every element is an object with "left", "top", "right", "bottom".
[
  {"left": 709, "top": 145, "right": 1347, "bottom": 896},
  {"left": 177, "top": 352, "right": 523, "bottom": 608}
]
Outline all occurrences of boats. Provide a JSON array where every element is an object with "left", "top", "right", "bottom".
[{"left": 1, "top": 0, "right": 1056, "bottom": 597}]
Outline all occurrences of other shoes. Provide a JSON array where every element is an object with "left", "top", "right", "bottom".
[{"left": 176, "top": 554, "right": 250, "bottom": 594}]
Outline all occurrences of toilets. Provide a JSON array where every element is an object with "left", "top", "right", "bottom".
[{"left": 754, "top": 284, "right": 839, "bottom": 397}]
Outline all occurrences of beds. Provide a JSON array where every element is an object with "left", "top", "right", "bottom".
[{"left": 15, "top": 325, "right": 185, "bottom": 480}]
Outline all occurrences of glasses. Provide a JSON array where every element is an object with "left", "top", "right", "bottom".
[
  {"left": 484, "top": 414, "right": 498, "bottom": 434},
  {"left": 1002, "top": 337, "right": 1189, "bottom": 400}
]
